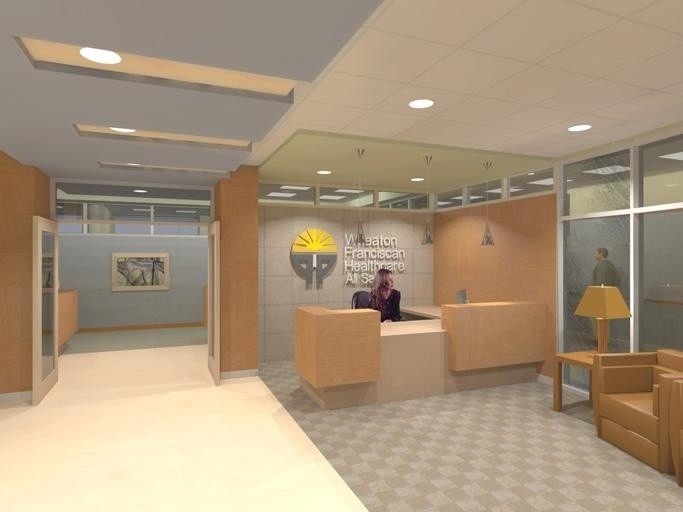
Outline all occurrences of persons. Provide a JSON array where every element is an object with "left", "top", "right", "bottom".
[
  {"left": 591, "top": 245, "right": 621, "bottom": 288},
  {"left": 364, "top": 267, "right": 402, "bottom": 323}
]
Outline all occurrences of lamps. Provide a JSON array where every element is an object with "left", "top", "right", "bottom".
[
  {"left": 481, "top": 161, "right": 494, "bottom": 245},
  {"left": 421, "top": 156, "right": 434, "bottom": 244},
  {"left": 355, "top": 149, "right": 366, "bottom": 244},
  {"left": 574, "top": 285, "right": 632, "bottom": 358}
]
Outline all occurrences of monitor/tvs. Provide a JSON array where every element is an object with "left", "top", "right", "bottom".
[{"left": 455, "top": 289, "right": 470, "bottom": 304}]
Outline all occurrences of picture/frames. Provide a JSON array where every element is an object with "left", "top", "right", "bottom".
[
  {"left": 111, "top": 253, "right": 169, "bottom": 291},
  {"left": 43, "top": 252, "right": 54, "bottom": 294}
]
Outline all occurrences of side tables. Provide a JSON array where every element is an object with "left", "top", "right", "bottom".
[{"left": 552, "top": 351, "right": 597, "bottom": 410}]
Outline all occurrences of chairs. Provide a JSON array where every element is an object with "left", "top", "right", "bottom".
[
  {"left": 591, "top": 348, "right": 683, "bottom": 474},
  {"left": 351, "top": 291, "right": 370, "bottom": 309},
  {"left": 669, "top": 378, "right": 683, "bottom": 487}
]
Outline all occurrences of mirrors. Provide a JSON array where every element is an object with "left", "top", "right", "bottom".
[
  {"left": 32, "top": 214, "right": 58, "bottom": 407},
  {"left": 208, "top": 220, "right": 221, "bottom": 386}
]
